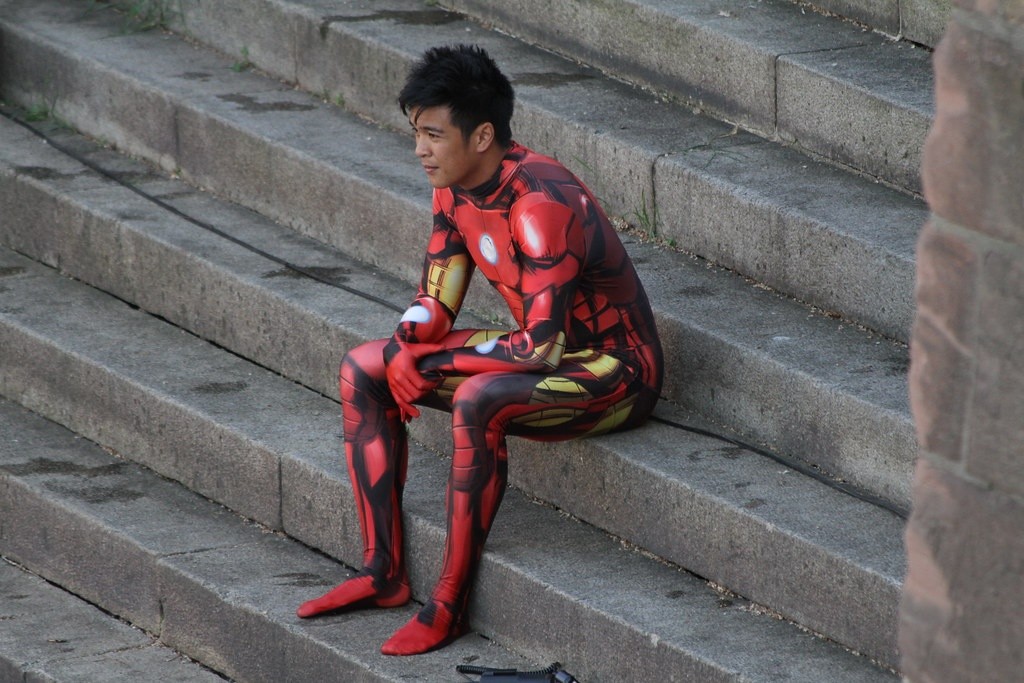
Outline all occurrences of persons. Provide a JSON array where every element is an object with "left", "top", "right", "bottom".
[{"left": 297, "top": 44, "right": 663, "bottom": 655}]
